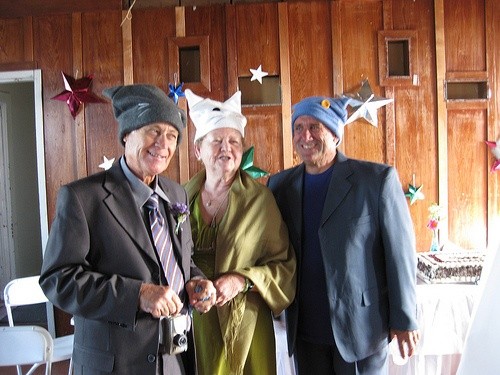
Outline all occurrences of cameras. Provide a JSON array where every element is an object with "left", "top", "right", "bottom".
[{"left": 161, "top": 313, "right": 187, "bottom": 355}]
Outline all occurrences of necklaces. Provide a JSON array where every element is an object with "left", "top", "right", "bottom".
[{"left": 205, "top": 185, "right": 230, "bottom": 226}]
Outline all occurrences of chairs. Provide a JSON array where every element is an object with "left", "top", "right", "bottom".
[
  {"left": 3, "top": 275, "right": 75, "bottom": 375},
  {"left": 0, "top": 325, "right": 53, "bottom": 375}
]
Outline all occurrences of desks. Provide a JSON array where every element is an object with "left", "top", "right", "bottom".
[{"left": 273, "top": 277, "right": 479, "bottom": 375}]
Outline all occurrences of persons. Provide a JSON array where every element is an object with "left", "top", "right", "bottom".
[
  {"left": 181, "top": 89, "right": 296, "bottom": 375},
  {"left": 267, "top": 97, "right": 419, "bottom": 375},
  {"left": 39, "top": 84, "right": 215, "bottom": 375}
]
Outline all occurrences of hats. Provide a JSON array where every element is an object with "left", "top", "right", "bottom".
[
  {"left": 291, "top": 97, "right": 350, "bottom": 146},
  {"left": 111, "top": 84, "right": 184, "bottom": 144},
  {"left": 183, "top": 88, "right": 247, "bottom": 141}
]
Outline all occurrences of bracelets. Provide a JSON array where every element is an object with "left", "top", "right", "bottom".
[{"left": 242, "top": 277, "right": 254, "bottom": 293}]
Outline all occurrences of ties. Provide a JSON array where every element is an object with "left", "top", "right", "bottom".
[{"left": 144, "top": 194, "right": 190, "bottom": 335}]
